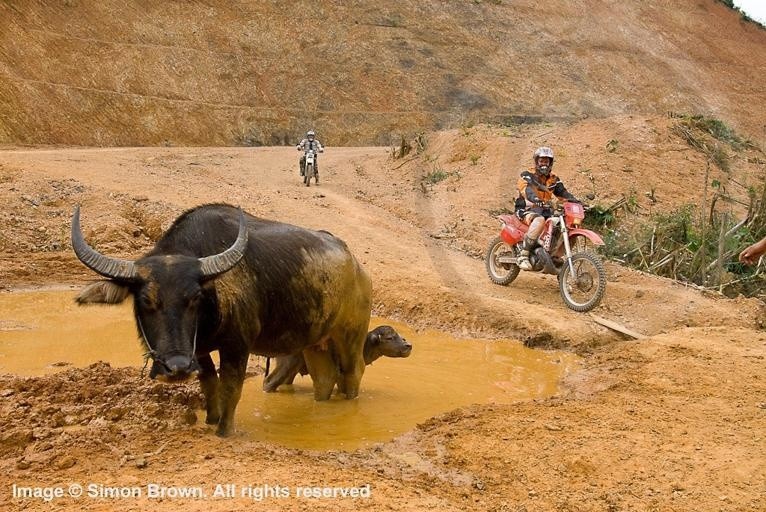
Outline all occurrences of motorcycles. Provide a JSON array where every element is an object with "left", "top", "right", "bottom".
[
  {"left": 488, "top": 198, "right": 607, "bottom": 312},
  {"left": 296, "top": 144, "right": 325, "bottom": 187}
]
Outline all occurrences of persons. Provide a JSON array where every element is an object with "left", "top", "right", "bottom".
[
  {"left": 738, "top": 237, "right": 765, "bottom": 268},
  {"left": 297, "top": 130, "right": 324, "bottom": 186},
  {"left": 514, "top": 146, "right": 578, "bottom": 270}
]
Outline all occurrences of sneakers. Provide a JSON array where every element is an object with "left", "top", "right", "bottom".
[{"left": 515, "top": 249, "right": 532, "bottom": 271}]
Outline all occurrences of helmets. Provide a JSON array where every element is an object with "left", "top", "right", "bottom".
[
  {"left": 307, "top": 131, "right": 315, "bottom": 136},
  {"left": 533, "top": 147, "right": 554, "bottom": 175}
]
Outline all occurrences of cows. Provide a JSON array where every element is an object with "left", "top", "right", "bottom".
[
  {"left": 69, "top": 201, "right": 374, "bottom": 439},
  {"left": 262, "top": 324, "right": 412, "bottom": 394}
]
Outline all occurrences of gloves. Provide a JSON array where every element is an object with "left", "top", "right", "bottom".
[{"left": 534, "top": 197, "right": 545, "bottom": 208}]
